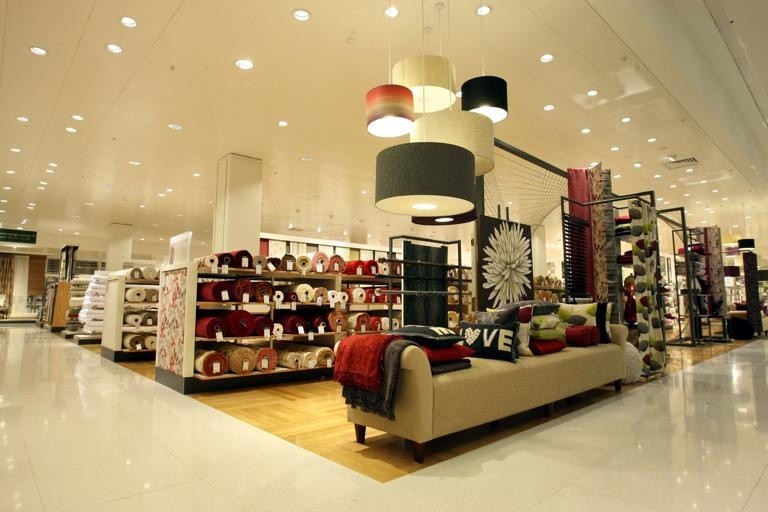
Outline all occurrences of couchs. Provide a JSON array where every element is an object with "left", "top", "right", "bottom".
[{"left": 335, "top": 323, "right": 628, "bottom": 463}]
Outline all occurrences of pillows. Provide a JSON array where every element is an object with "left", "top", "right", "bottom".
[{"left": 383, "top": 299, "right": 613, "bottom": 374}]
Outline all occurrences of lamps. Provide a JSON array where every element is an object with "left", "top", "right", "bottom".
[{"left": 366, "top": 0, "right": 508, "bottom": 225}]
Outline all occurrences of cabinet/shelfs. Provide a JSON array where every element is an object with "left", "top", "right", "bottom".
[
  {"left": 62, "top": 261, "right": 565, "bottom": 396},
  {"left": 672, "top": 227, "right": 731, "bottom": 344}
]
